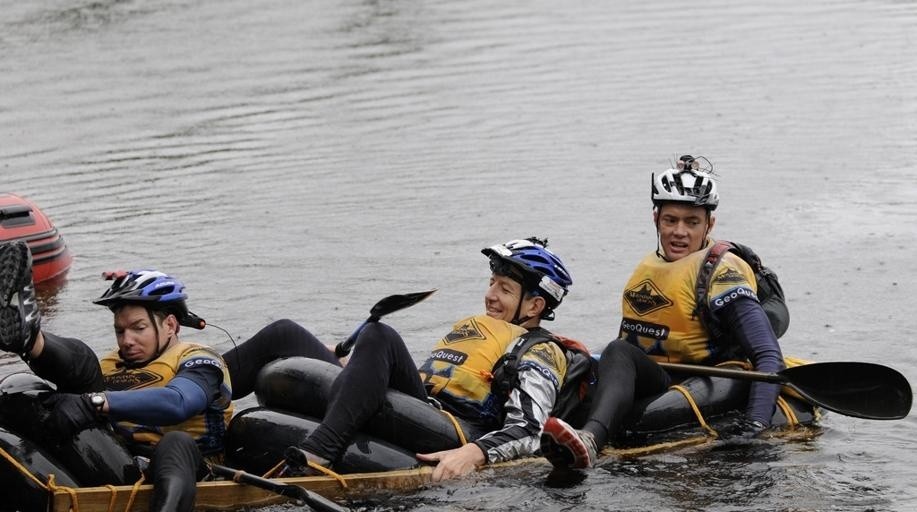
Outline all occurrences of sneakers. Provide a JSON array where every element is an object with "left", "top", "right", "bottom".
[
  {"left": 539, "top": 417, "right": 598, "bottom": 470},
  {"left": 0, "top": 239, "right": 41, "bottom": 354},
  {"left": 272, "top": 447, "right": 333, "bottom": 476}
]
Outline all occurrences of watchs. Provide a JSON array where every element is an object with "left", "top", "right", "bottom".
[{"left": 89, "top": 392, "right": 105, "bottom": 414}]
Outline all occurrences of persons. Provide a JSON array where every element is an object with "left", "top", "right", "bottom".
[
  {"left": 0, "top": 242, "right": 229, "bottom": 512},
  {"left": 537, "top": 168, "right": 787, "bottom": 477},
  {"left": 221, "top": 238, "right": 573, "bottom": 486}
]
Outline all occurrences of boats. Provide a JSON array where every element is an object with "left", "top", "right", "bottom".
[
  {"left": 0, "top": 191, "right": 75, "bottom": 280},
  {"left": 0, "top": 356, "right": 826, "bottom": 512}
]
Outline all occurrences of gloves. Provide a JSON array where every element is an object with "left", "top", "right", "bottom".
[{"left": 37, "top": 391, "right": 97, "bottom": 451}]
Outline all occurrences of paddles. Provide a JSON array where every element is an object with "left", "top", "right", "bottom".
[
  {"left": 655, "top": 359, "right": 913, "bottom": 420},
  {"left": 336, "top": 289, "right": 437, "bottom": 358},
  {"left": 131, "top": 441, "right": 356, "bottom": 512}
]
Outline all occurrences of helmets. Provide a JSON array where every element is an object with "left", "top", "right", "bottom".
[
  {"left": 480, "top": 239, "right": 573, "bottom": 310},
  {"left": 653, "top": 168, "right": 720, "bottom": 211},
  {"left": 92, "top": 266, "right": 189, "bottom": 306}
]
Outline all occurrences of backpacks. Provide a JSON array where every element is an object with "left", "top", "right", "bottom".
[
  {"left": 695, "top": 240, "right": 790, "bottom": 361},
  {"left": 489, "top": 332, "right": 597, "bottom": 431}
]
